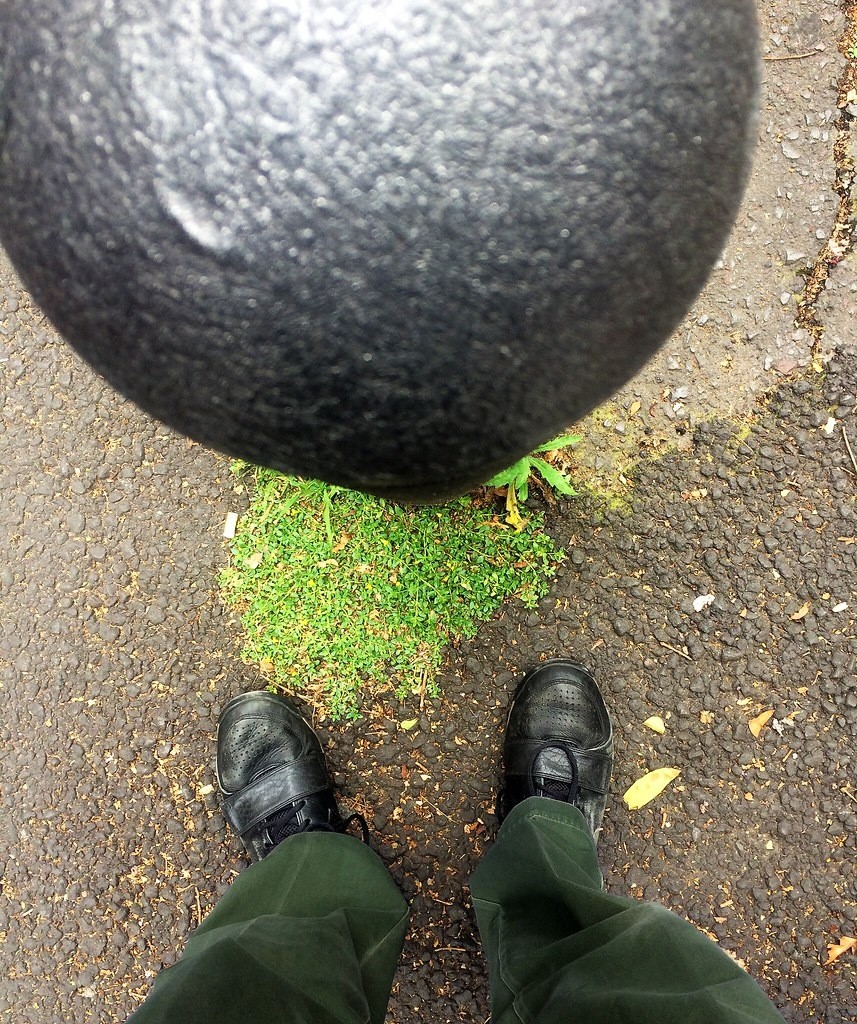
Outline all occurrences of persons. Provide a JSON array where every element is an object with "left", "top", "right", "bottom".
[{"left": 124, "top": 657, "right": 789, "bottom": 1024}]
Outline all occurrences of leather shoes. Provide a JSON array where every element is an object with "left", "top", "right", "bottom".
[
  {"left": 494, "top": 657, "right": 615, "bottom": 844},
  {"left": 214, "top": 690, "right": 370, "bottom": 862}
]
